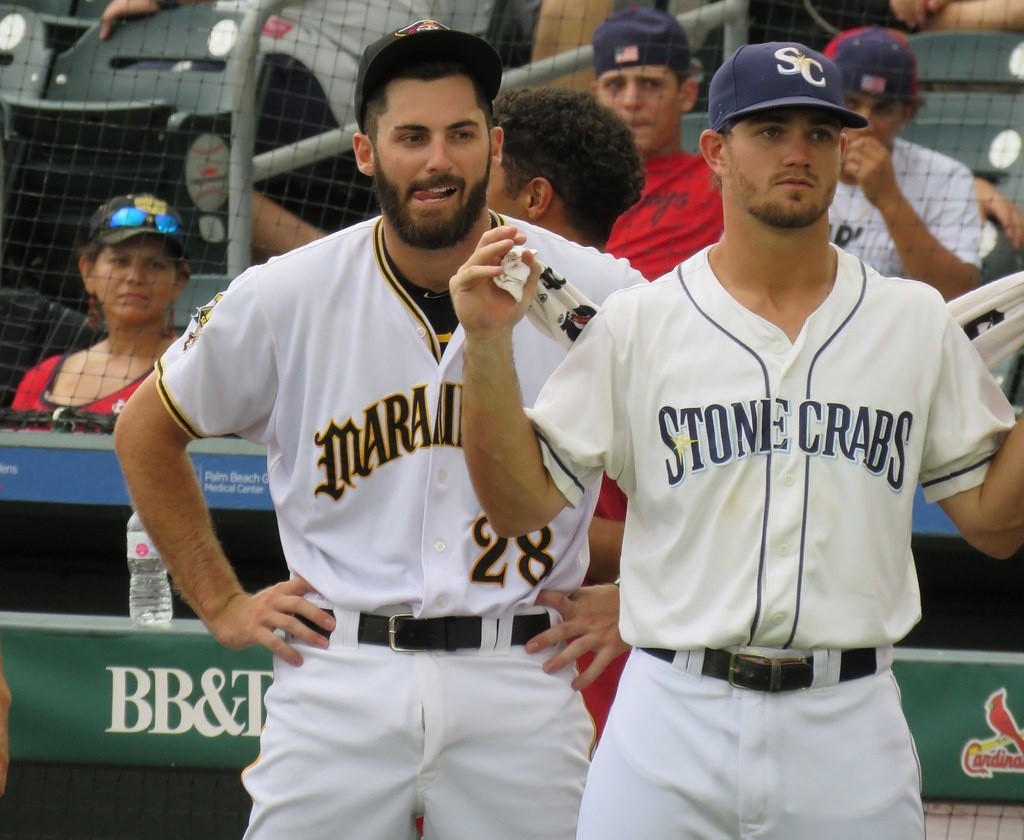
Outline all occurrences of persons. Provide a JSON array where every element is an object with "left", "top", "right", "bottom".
[
  {"left": 114, "top": 25, "right": 654, "bottom": 838},
  {"left": 2, "top": 191, "right": 201, "bottom": 434},
  {"left": 810, "top": 26, "right": 996, "bottom": 301},
  {"left": 448, "top": 43, "right": 1024, "bottom": 839},
  {"left": 223, "top": 94, "right": 646, "bottom": 761},
  {"left": 586, "top": 12, "right": 1023, "bottom": 281}
]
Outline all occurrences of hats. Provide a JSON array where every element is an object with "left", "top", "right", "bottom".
[
  {"left": 821, "top": 27, "right": 922, "bottom": 102},
  {"left": 353, "top": 19, "right": 504, "bottom": 137},
  {"left": 593, "top": 7, "right": 691, "bottom": 88},
  {"left": 86, "top": 192, "right": 188, "bottom": 263},
  {"left": 707, "top": 41, "right": 870, "bottom": 132}
]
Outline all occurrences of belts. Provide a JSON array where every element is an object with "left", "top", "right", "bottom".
[
  {"left": 291, "top": 607, "right": 551, "bottom": 654},
  {"left": 637, "top": 640, "right": 876, "bottom": 693}
]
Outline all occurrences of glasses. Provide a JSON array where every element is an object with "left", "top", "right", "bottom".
[{"left": 97, "top": 206, "right": 183, "bottom": 239}]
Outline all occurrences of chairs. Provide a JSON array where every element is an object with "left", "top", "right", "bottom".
[{"left": 0, "top": 0, "right": 1024, "bottom": 214}]
